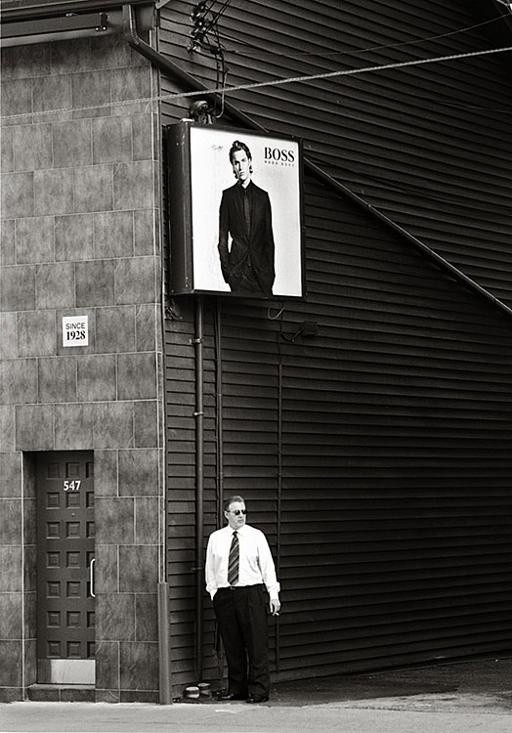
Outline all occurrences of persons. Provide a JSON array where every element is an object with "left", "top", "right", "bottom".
[
  {"left": 204, "top": 494, "right": 283, "bottom": 704},
  {"left": 217, "top": 138, "right": 276, "bottom": 293}
]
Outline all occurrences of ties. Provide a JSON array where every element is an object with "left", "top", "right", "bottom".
[
  {"left": 228, "top": 531, "right": 239, "bottom": 584},
  {"left": 244, "top": 191, "right": 253, "bottom": 237}
]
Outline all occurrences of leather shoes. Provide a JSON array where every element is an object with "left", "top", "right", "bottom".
[
  {"left": 218, "top": 693, "right": 250, "bottom": 700},
  {"left": 247, "top": 696, "right": 269, "bottom": 703}
]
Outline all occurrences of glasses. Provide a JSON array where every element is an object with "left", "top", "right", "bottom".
[{"left": 229, "top": 510, "right": 247, "bottom": 514}]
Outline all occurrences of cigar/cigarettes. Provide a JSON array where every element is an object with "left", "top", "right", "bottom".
[{"left": 274, "top": 611, "right": 280, "bottom": 616}]
